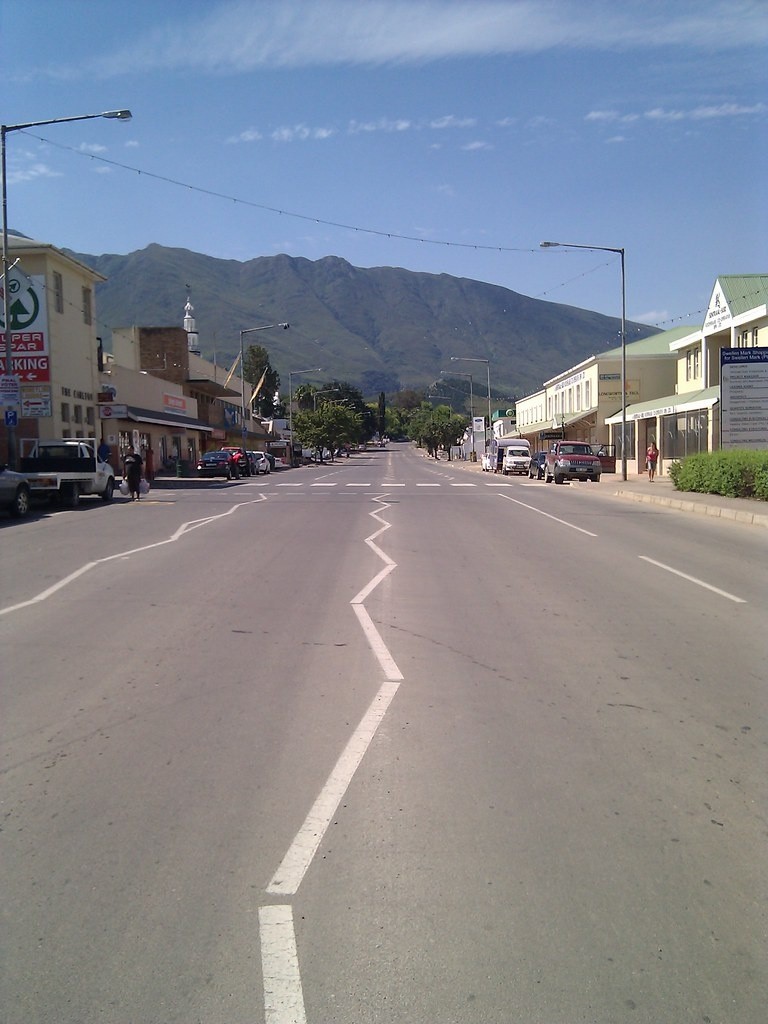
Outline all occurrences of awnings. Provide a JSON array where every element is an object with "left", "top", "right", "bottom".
[{"left": 127, "top": 406, "right": 214, "bottom": 435}]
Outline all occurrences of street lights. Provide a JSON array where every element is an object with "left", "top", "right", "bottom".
[
  {"left": 239, "top": 322, "right": 290, "bottom": 450},
  {"left": 440, "top": 371, "right": 474, "bottom": 462},
  {"left": 313, "top": 389, "right": 339, "bottom": 411},
  {"left": 288, "top": 368, "right": 322, "bottom": 468},
  {"left": 429, "top": 395, "right": 451, "bottom": 417},
  {"left": 450, "top": 357, "right": 492, "bottom": 452},
  {"left": 539, "top": 241, "right": 628, "bottom": 482},
  {"left": 416, "top": 409, "right": 433, "bottom": 420},
  {"left": 2, "top": 108, "right": 134, "bottom": 461}
]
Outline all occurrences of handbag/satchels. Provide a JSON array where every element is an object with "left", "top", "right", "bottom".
[
  {"left": 119, "top": 479, "right": 129, "bottom": 495},
  {"left": 139, "top": 478, "right": 150, "bottom": 494}
]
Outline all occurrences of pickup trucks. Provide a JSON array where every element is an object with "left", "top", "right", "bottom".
[{"left": 15, "top": 437, "right": 116, "bottom": 507}]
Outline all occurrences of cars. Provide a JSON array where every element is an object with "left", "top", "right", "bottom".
[
  {"left": 246, "top": 450, "right": 259, "bottom": 475},
  {"left": 543, "top": 441, "right": 617, "bottom": 484},
  {"left": 528, "top": 451, "right": 548, "bottom": 480},
  {"left": 197, "top": 451, "right": 236, "bottom": 480},
  {"left": 380, "top": 443, "right": 385, "bottom": 447},
  {"left": 481, "top": 452, "right": 490, "bottom": 472},
  {"left": 220, "top": 447, "right": 249, "bottom": 477},
  {"left": 253, "top": 451, "right": 270, "bottom": 474},
  {"left": 501, "top": 445, "right": 532, "bottom": 475},
  {"left": 359, "top": 445, "right": 366, "bottom": 451},
  {"left": 0, "top": 462, "right": 30, "bottom": 519}
]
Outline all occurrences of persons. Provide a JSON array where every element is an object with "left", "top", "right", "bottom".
[
  {"left": 345, "top": 441, "right": 352, "bottom": 457},
  {"left": 232, "top": 450, "right": 244, "bottom": 480},
  {"left": 166, "top": 455, "right": 173, "bottom": 471},
  {"left": 645, "top": 441, "right": 660, "bottom": 482},
  {"left": 140, "top": 444, "right": 154, "bottom": 483},
  {"left": 123, "top": 446, "right": 145, "bottom": 503}
]
